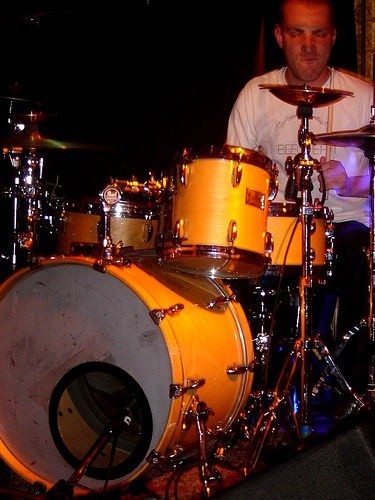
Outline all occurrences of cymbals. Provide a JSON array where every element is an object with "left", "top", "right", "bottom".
[
  {"left": 0, "top": 127, "right": 78, "bottom": 152},
  {"left": 258, "top": 83, "right": 355, "bottom": 108},
  {"left": 0, "top": 96, "right": 26, "bottom": 102},
  {"left": 308, "top": 124, "right": 375, "bottom": 147}
]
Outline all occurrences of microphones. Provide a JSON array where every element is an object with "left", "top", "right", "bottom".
[{"left": 284, "top": 171, "right": 299, "bottom": 201}]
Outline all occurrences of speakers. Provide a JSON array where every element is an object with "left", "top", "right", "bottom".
[{"left": 218, "top": 421, "right": 375, "bottom": 500}]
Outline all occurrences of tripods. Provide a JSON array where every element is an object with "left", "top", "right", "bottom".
[{"left": 238, "top": 104, "right": 375, "bottom": 476}]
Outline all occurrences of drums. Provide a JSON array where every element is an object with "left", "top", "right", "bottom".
[
  {"left": 54, "top": 190, "right": 166, "bottom": 258},
  {"left": 265, "top": 200, "right": 328, "bottom": 267},
  {"left": 0, "top": 252, "right": 257, "bottom": 498},
  {"left": 155, "top": 141, "right": 278, "bottom": 281}
]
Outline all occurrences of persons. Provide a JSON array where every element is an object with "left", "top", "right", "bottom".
[{"left": 224, "top": 0, "right": 375, "bottom": 431}]
